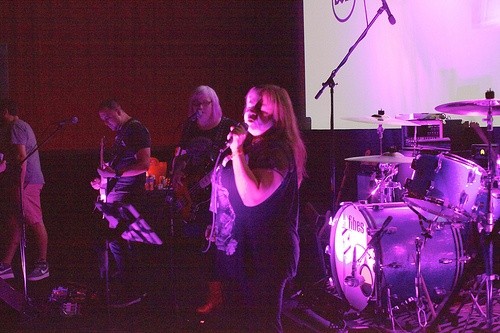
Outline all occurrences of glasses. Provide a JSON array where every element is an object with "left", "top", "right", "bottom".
[{"left": 192, "top": 100, "right": 211, "bottom": 107}]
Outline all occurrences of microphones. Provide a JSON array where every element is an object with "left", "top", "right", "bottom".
[
  {"left": 187, "top": 110, "right": 202, "bottom": 121},
  {"left": 344, "top": 275, "right": 359, "bottom": 287},
  {"left": 56, "top": 117, "right": 78, "bottom": 126},
  {"left": 219, "top": 122, "right": 249, "bottom": 153},
  {"left": 381, "top": 0, "right": 396, "bottom": 25}
]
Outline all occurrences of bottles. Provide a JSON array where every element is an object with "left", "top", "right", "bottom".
[
  {"left": 158, "top": 176, "right": 163, "bottom": 190},
  {"left": 147, "top": 176, "right": 154, "bottom": 190}
]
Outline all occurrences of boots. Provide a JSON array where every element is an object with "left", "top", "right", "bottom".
[{"left": 195, "top": 282, "right": 224, "bottom": 315}]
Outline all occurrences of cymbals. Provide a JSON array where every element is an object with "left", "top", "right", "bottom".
[
  {"left": 343, "top": 151, "right": 416, "bottom": 164},
  {"left": 341, "top": 115, "right": 422, "bottom": 127},
  {"left": 434, "top": 98, "right": 500, "bottom": 117}
]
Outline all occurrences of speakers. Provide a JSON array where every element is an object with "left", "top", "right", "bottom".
[{"left": 0, "top": 277, "right": 26, "bottom": 324}]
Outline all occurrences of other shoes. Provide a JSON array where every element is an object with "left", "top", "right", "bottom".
[{"left": 110, "top": 290, "right": 141, "bottom": 308}]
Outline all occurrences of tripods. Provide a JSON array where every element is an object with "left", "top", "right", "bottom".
[{"left": 450, "top": 105, "right": 500, "bottom": 329}]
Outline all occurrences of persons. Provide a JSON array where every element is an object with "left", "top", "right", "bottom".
[
  {"left": 171, "top": 85, "right": 253, "bottom": 315},
  {"left": 90, "top": 100, "right": 151, "bottom": 284},
  {"left": 203, "top": 84, "right": 306, "bottom": 333},
  {"left": 0, "top": 99, "right": 49, "bottom": 280}
]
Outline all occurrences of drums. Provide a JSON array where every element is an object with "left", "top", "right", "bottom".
[
  {"left": 402, "top": 150, "right": 488, "bottom": 221},
  {"left": 328, "top": 200, "right": 466, "bottom": 314},
  {"left": 471, "top": 188, "right": 500, "bottom": 222}
]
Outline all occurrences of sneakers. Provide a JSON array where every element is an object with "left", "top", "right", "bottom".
[
  {"left": 26, "top": 263, "right": 49, "bottom": 282},
  {"left": 0, "top": 263, "right": 15, "bottom": 279}
]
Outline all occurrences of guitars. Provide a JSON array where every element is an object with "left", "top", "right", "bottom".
[
  {"left": 97, "top": 131, "right": 107, "bottom": 220},
  {"left": 172, "top": 142, "right": 253, "bottom": 224}
]
